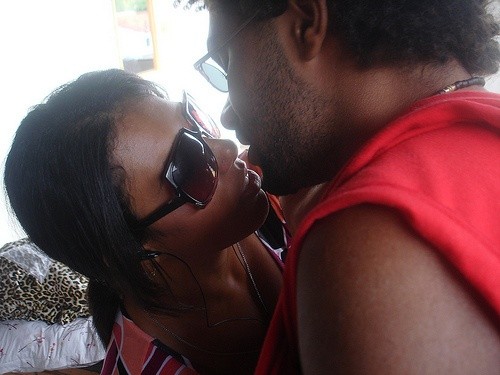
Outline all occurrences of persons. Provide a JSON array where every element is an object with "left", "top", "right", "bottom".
[
  {"left": 7, "top": 69, "right": 295, "bottom": 374},
  {"left": 202, "top": 1, "right": 499, "bottom": 375}
]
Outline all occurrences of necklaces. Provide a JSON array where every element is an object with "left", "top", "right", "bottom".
[
  {"left": 149, "top": 240, "right": 271, "bottom": 368},
  {"left": 428, "top": 75, "right": 489, "bottom": 94}
]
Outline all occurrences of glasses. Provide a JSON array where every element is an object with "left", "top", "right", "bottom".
[
  {"left": 131, "top": 87, "right": 220, "bottom": 227},
  {"left": 193, "top": 4, "right": 276, "bottom": 93}
]
